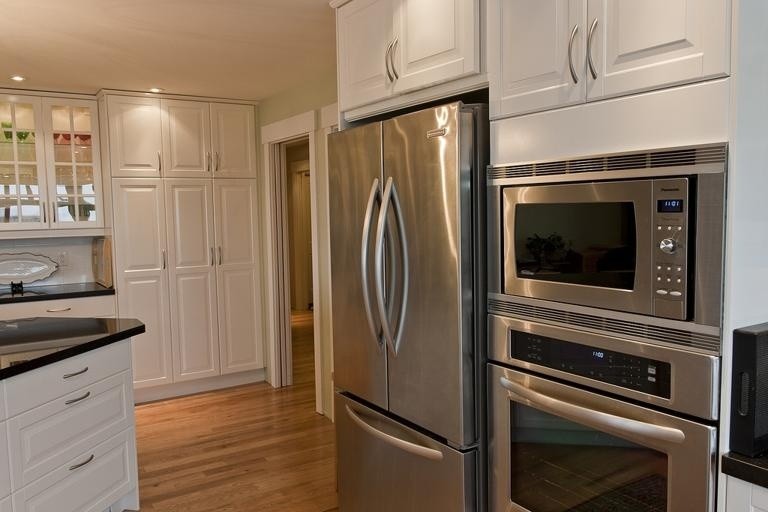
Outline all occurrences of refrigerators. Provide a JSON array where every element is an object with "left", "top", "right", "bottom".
[{"left": 328, "top": 103, "right": 486, "bottom": 512}]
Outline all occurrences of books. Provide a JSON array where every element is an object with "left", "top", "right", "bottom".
[{"left": 92, "top": 238, "right": 113, "bottom": 288}]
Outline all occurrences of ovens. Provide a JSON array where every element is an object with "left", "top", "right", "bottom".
[{"left": 489, "top": 312, "right": 718, "bottom": 512}]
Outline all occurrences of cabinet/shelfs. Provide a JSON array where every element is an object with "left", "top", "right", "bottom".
[
  {"left": 163, "top": 178, "right": 266, "bottom": 400},
  {"left": 0, "top": 338, "right": 140, "bottom": 512},
  {"left": 1, "top": 295, "right": 117, "bottom": 317},
  {"left": 0, "top": 89, "right": 105, "bottom": 239},
  {"left": 718, "top": 470, "right": 768, "bottom": 512},
  {"left": 100, "top": 178, "right": 172, "bottom": 405},
  {"left": 330, "top": 0, "right": 487, "bottom": 128},
  {"left": 489, "top": 1, "right": 768, "bottom": 122},
  {"left": 98, "top": 87, "right": 165, "bottom": 178},
  {"left": 161, "top": 92, "right": 259, "bottom": 178}
]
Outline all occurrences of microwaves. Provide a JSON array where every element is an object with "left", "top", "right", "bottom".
[{"left": 491, "top": 143, "right": 729, "bottom": 317}]
